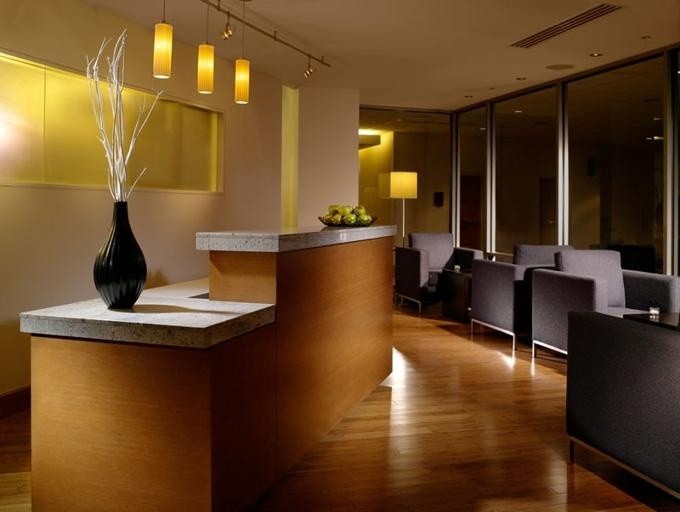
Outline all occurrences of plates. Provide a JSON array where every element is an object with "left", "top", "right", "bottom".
[{"left": 318, "top": 216, "right": 379, "bottom": 227}]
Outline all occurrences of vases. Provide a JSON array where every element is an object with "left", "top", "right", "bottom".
[{"left": 93, "top": 198, "right": 149, "bottom": 312}]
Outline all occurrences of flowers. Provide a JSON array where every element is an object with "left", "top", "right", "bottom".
[{"left": 87, "top": 29, "right": 168, "bottom": 201}]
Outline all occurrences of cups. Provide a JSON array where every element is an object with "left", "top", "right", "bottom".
[
  {"left": 454, "top": 265, "right": 460, "bottom": 273},
  {"left": 649, "top": 307, "right": 660, "bottom": 322}
]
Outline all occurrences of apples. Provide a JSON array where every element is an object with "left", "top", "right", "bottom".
[
  {"left": 354, "top": 205, "right": 366, "bottom": 216},
  {"left": 341, "top": 205, "right": 354, "bottom": 216},
  {"left": 328, "top": 204, "right": 344, "bottom": 217},
  {"left": 343, "top": 214, "right": 357, "bottom": 224},
  {"left": 368, "top": 215, "right": 372, "bottom": 223},
  {"left": 323, "top": 214, "right": 333, "bottom": 222},
  {"left": 331, "top": 213, "right": 342, "bottom": 224},
  {"left": 357, "top": 215, "right": 369, "bottom": 223}
]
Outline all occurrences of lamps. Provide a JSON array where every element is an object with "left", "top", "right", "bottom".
[
  {"left": 152, "top": 0, "right": 173, "bottom": 81},
  {"left": 196, "top": 0, "right": 216, "bottom": 93},
  {"left": 390, "top": 170, "right": 418, "bottom": 246},
  {"left": 234, "top": 0, "right": 249, "bottom": 103}
]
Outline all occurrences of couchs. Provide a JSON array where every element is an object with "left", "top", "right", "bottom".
[
  {"left": 532, "top": 248, "right": 680, "bottom": 356},
  {"left": 396, "top": 232, "right": 484, "bottom": 315},
  {"left": 566, "top": 309, "right": 680, "bottom": 512},
  {"left": 473, "top": 244, "right": 574, "bottom": 350}
]
